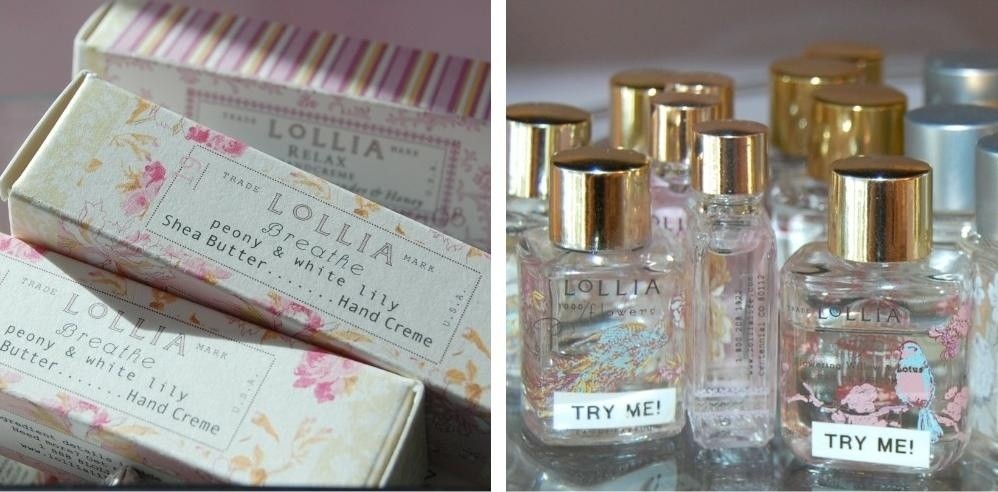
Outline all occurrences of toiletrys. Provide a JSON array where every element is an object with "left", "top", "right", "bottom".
[{"left": 506, "top": 37, "right": 998, "bottom": 478}]
